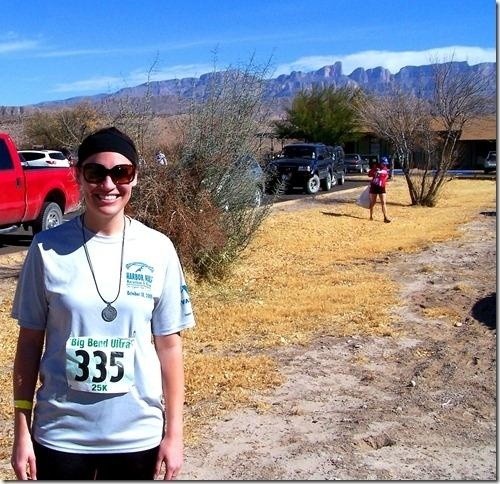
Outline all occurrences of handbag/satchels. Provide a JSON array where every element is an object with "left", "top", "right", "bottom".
[{"left": 356, "top": 184, "right": 371, "bottom": 208}]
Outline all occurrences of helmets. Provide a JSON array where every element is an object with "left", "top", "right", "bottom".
[{"left": 380, "top": 156, "right": 390, "bottom": 164}]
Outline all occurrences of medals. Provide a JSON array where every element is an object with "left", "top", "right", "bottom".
[{"left": 101, "top": 304, "right": 118, "bottom": 322}]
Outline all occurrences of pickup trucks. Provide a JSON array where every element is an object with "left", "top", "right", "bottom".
[{"left": 0, "top": 132, "right": 81, "bottom": 235}]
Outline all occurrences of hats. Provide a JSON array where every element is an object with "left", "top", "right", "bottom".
[{"left": 78, "top": 127, "right": 138, "bottom": 168}]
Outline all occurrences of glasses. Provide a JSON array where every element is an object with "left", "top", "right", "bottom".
[{"left": 78, "top": 163, "right": 136, "bottom": 184}]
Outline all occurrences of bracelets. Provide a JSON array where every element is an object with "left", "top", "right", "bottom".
[{"left": 13, "top": 399, "right": 32, "bottom": 410}]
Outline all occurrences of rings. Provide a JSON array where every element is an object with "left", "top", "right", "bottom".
[{"left": 173, "top": 475, "right": 177, "bottom": 477}]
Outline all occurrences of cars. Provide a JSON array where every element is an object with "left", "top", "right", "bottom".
[{"left": 222, "top": 155, "right": 265, "bottom": 211}]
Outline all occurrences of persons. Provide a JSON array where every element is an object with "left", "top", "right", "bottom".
[
  {"left": 8, "top": 124, "right": 198, "bottom": 481},
  {"left": 153, "top": 149, "right": 167, "bottom": 166},
  {"left": 136, "top": 149, "right": 146, "bottom": 164},
  {"left": 367, "top": 156, "right": 391, "bottom": 223}
]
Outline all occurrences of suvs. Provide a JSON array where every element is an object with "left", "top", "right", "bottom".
[
  {"left": 264, "top": 143, "right": 363, "bottom": 194},
  {"left": 484, "top": 151, "right": 496, "bottom": 174}
]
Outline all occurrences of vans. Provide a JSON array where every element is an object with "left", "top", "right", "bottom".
[{"left": 18, "top": 150, "right": 70, "bottom": 167}]
